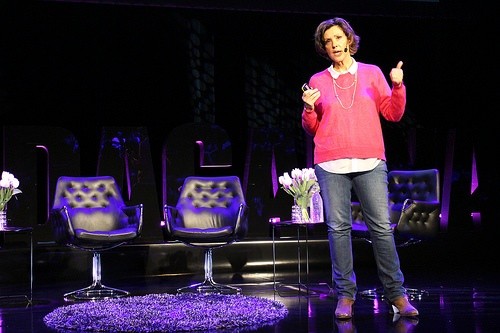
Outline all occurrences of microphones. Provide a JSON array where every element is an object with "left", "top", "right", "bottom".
[{"left": 344, "top": 43, "right": 347, "bottom": 52}]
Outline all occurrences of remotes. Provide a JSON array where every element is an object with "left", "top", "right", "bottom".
[{"left": 302, "top": 83, "right": 311, "bottom": 92}]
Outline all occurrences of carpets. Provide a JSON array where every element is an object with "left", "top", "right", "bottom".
[{"left": 42, "top": 295, "right": 292, "bottom": 333}]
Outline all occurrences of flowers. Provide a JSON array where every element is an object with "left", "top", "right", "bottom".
[
  {"left": 0, "top": 171, "right": 23, "bottom": 212},
  {"left": 280, "top": 167, "right": 321, "bottom": 222}
]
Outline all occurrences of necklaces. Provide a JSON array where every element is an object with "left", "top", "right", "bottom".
[{"left": 332, "top": 72, "right": 358, "bottom": 110}]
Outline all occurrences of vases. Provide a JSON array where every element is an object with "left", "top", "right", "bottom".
[
  {"left": 0, "top": 210, "right": 7, "bottom": 228},
  {"left": 295, "top": 199, "right": 312, "bottom": 223}
]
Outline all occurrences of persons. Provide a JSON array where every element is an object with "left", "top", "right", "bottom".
[{"left": 301, "top": 18, "right": 419, "bottom": 317}]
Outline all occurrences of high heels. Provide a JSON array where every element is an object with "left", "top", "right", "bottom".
[
  {"left": 385, "top": 295, "right": 419, "bottom": 316},
  {"left": 335, "top": 299, "right": 354, "bottom": 319}
]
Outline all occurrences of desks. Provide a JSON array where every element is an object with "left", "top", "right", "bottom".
[
  {"left": 270, "top": 221, "right": 333, "bottom": 298},
  {"left": 0, "top": 224, "right": 34, "bottom": 310}
]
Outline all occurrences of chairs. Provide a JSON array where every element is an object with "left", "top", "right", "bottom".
[
  {"left": 49, "top": 175, "right": 146, "bottom": 303},
  {"left": 349, "top": 167, "right": 444, "bottom": 305},
  {"left": 160, "top": 174, "right": 248, "bottom": 296}
]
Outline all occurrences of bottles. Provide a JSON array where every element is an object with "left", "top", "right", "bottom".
[{"left": 312, "top": 192, "right": 324, "bottom": 223}]
